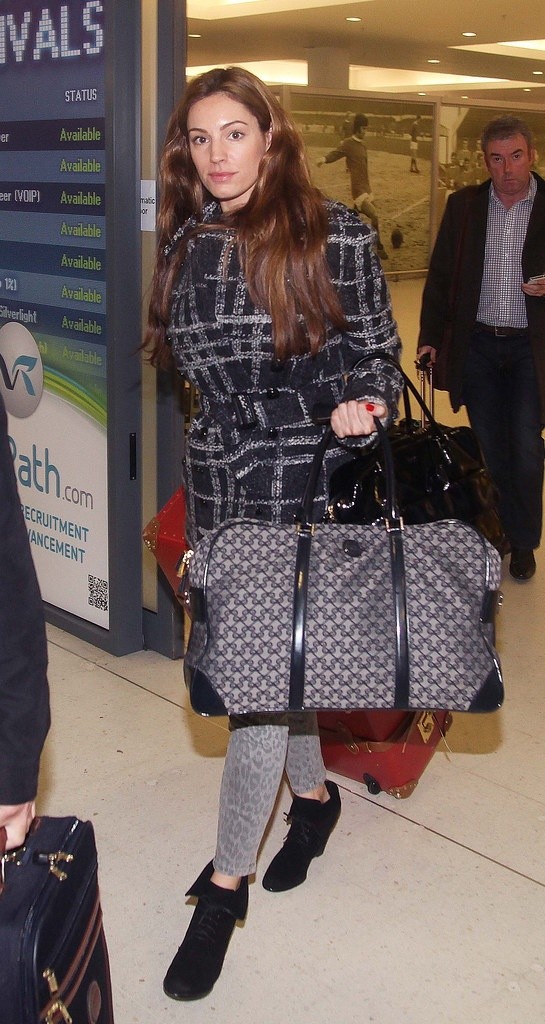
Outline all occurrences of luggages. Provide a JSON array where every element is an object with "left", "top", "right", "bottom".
[{"left": 139, "top": 486, "right": 454, "bottom": 800}]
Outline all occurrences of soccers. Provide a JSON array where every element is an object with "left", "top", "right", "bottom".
[{"left": 392, "top": 226, "right": 403, "bottom": 252}]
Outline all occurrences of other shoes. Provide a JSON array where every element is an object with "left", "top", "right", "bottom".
[
  {"left": 163, "top": 857, "right": 249, "bottom": 1001},
  {"left": 376, "top": 243, "right": 389, "bottom": 261},
  {"left": 262, "top": 780, "right": 342, "bottom": 892}
]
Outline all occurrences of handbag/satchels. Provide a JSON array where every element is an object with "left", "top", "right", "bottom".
[
  {"left": 176, "top": 417, "right": 505, "bottom": 717},
  {"left": 0, "top": 815, "right": 114, "bottom": 1024},
  {"left": 327, "top": 351, "right": 504, "bottom": 542}
]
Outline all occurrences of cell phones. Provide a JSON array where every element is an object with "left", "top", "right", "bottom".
[{"left": 527, "top": 275, "right": 545, "bottom": 285}]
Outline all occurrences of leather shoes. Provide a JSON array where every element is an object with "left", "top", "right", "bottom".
[{"left": 509, "top": 543, "right": 536, "bottom": 580}]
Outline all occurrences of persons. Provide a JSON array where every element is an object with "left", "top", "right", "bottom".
[
  {"left": 147, "top": 68, "right": 404, "bottom": 1000},
  {"left": 340, "top": 111, "right": 354, "bottom": 172},
  {"left": 317, "top": 114, "right": 389, "bottom": 260},
  {"left": 414, "top": 116, "right": 545, "bottom": 581},
  {"left": 0, "top": 391, "right": 52, "bottom": 847},
  {"left": 409, "top": 115, "right": 421, "bottom": 173},
  {"left": 445, "top": 138, "right": 490, "bottom": 186}
]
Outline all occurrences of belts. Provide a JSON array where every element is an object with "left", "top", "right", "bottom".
[{"left": 472, "top": 321, "right": 530, "bottom": 337}]
